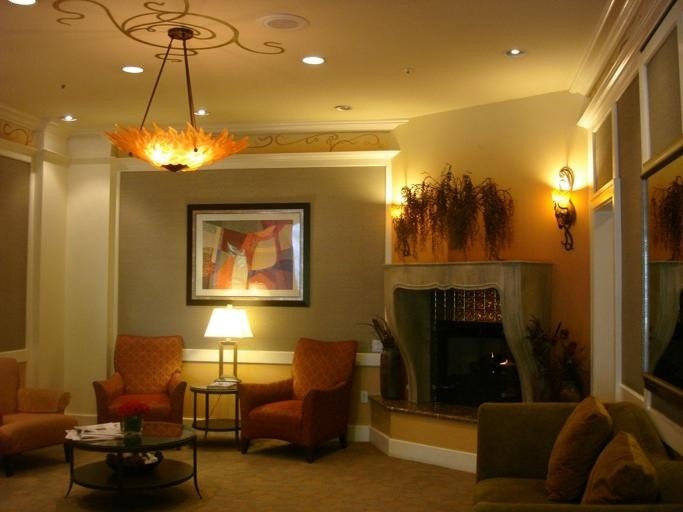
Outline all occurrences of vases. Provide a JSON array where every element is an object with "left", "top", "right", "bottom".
[{"left": 124, "top": 416, "right": 142, "bottom": 432}]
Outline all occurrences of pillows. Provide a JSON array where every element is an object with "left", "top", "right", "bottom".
[{"left": 545, "top": 395, "right": 658, "bottom": 504}]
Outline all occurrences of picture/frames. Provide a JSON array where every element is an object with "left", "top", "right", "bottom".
[{"left": 186, "top": 202, "right": 311, "bottom": 308}]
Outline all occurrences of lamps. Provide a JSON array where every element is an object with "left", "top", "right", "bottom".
[
  {"left": 102, "top": 26, "right": 251, "bottom": 173},
  {"left": 203, "top": 304, "right": 254, "bottom": 383},
  {"left": 551, "top": 166, "right": 576, "bottom": 251}
]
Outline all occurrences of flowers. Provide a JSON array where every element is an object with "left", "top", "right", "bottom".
[{"left": 114, "top": 400, "right": 150, "bottom": 417}]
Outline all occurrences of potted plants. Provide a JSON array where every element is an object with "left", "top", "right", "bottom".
[
  {"left": 354, "top": 315, "right": 407, "bottom": 401},
  {"left": 391, "top": 161, "right": 516, "bottom": 264}
]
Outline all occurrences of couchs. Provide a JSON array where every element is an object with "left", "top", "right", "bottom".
[{"left": 470, "top": 402, "right": 683, "bottom": 512}]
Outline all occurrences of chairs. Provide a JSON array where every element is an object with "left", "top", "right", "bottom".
[
  {"left": 92, "top": 334, "right": 188, "bottom": 451},
  {"left": 0, "top": 357, "right": 78, "bottom": 478},
  {"left": 237, "top": 337, "right": 358, "bottom": 464}
]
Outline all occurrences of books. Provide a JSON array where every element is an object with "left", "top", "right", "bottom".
[{"left": 61, "top": 420, "right": 121, "bottom": 443}]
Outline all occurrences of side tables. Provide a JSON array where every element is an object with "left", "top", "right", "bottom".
[{"left": 189, "top": 385, "right": 241, "bottom": 446}]
